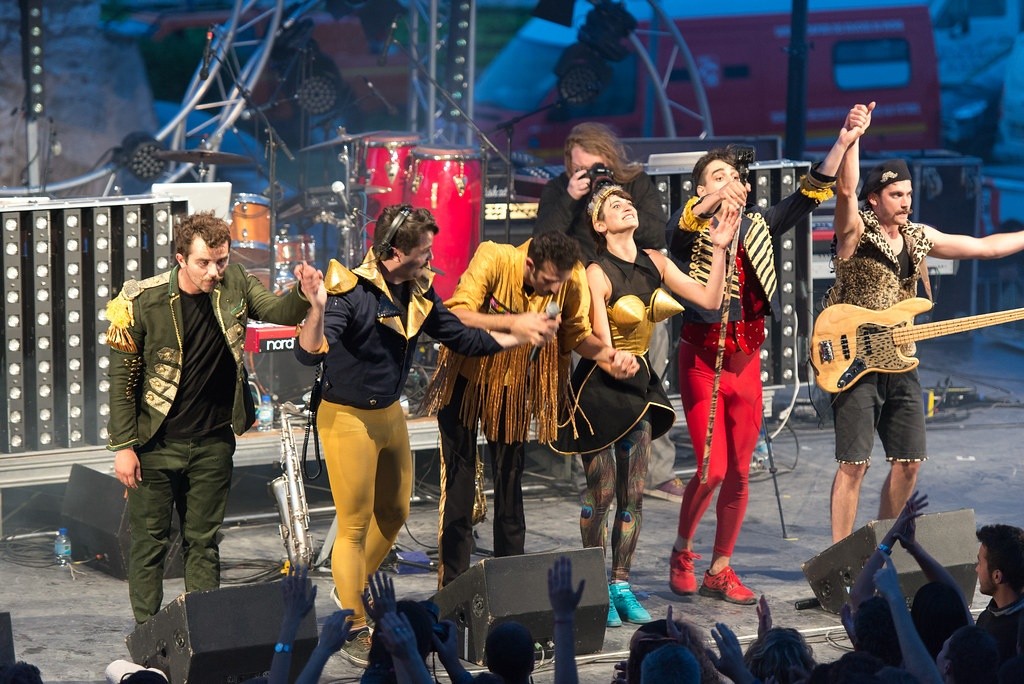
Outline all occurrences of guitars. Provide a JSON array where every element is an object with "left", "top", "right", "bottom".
[{"left": 810, "top": 295, "right": 1024, "bottom": 394}]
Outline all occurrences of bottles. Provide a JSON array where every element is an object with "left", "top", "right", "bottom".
[
  {"left": 55, "top": 527, "right": 71, "bottom": 557},
  {"left": 258, "top": 395, "right": 274, "bottom": 432},
  {"left": 755, "top": 429, "right": 773, "bottom": 454}
]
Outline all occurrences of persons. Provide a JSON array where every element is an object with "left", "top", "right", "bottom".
[
  {"left": 829, "top": 101, "right": 1024, "bottom": 545},
  {"left": 267, "top": 489, "right": 1024, "bottom": 684},
  {"left": 663, "top": 113, "right": 865, "bottom": 603},
  {"left": 533, "top": 121, "right": 688, "bottom": 504},
  {"left": 105, "top": 212, "right": 321, "bottom": 629},
  {"left": 541, "top": 184, "right": 741, "bottom": 627},
  {"left": 294, "top": 203, "right": 521, "bottom": 667},
  {"left": 427, "top": 228, "right": 641, "bottom": 593}
]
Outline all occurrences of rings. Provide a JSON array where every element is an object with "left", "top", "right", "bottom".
[{"left": 395, "top": 628, "right": 400, "bottom": 632}]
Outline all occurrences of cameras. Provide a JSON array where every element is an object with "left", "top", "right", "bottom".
[
  {"left": 416, "top": 600, "right": 448, "bottom": 652},
  {"left": 575, "top": 162, "right": 614, "bottom": 192}
]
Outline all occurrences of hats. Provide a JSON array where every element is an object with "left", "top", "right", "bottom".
[{"left": 857, "top": 158, "right": 911, "bottom": 199}]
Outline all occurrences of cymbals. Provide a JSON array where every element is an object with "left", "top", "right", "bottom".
[
  {"left": 299, "top": 128, "right": 384, "bottom": 152},
  {"left": 156, "top": 148, "right": 252, "bottom": 166}
]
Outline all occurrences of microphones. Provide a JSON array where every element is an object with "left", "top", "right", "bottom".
[
  {"left": 426, "top": 266, "right": 445, "bottom": 276},
  {"left": 529, "top": 301, "right": 559, "bottom": 361},
  {"left": 332, "top": 181, "right": 350, "bottom": 212},
  {"left": 240, "top": 102, "right": 274, "bottom": 119},
  {"left": 363, "top": 77, "right": 398, "bottom": 116},
  {"left": 378, "top": 14, "right": 399, "bottom": 65},
  {"left": 200, "top": 29, "right": 212, "bottom": 80}
]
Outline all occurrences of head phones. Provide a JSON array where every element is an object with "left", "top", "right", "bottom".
[{"left": 374, "top": 204, "right": 413, "bottom": 261}]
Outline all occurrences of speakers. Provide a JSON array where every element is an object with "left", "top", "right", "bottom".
[
  {"left": 61, "top": 464, "right": 184, "bottom": 581},
  {"left": 123, "top": 578, "right": 318, "bottom": 684},
  {"left": 801, "top": 509, "right": 977, "bottom": 614},
  {"left": 424, "top": 546, "right": 609, "bottom": 666}
]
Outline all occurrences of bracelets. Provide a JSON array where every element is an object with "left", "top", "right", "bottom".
[{"left": 553, "top": 620, "right": 573, "bottom": 624}]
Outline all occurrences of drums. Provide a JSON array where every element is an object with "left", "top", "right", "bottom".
[
  {"left": 273, "top": 235, "right": 317, "bottom": 269},
  {"left": 246, "top": 269, "right": 297, "bottom": 297},
  {"left": 355, "top": 130, "right": 484, "bottom": 305},
  {"left": 230, "top": 191, "right": 276, "bottom": 266}
]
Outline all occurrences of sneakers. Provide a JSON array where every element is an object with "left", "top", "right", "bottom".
[
  {"left": 697, "top": 564, "right": 755, "bottom": 606},
  {"left": 337, "top": 628, "right": 374, "bottom": 666},
  {"left": 610, "top": 582, "right": 651, "bottom": 623},
  {"left": 330, "top": 582, "right": 376, "bottom": 629},
  {"left": 605, "top": 582, "right": 621, "bottom": 629},
  {"left": 668, "top": 542, "right": 702, "bottom": 597},
  {"left": 643, "top": 477, "right": 687, "bottom": 503}
]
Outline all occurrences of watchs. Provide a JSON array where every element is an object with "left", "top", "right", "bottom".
[
  {"left": 274, "top": 642, "right": 293, "bottom": 653},
  {"left": 877, "top": 543, "right": 891, "bottom": 554}
]
